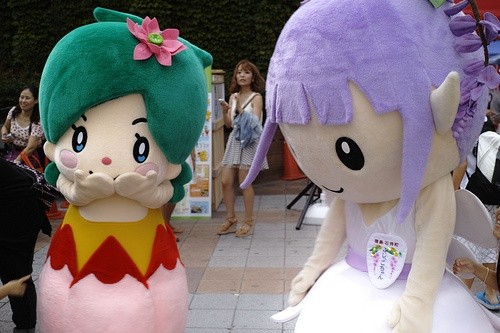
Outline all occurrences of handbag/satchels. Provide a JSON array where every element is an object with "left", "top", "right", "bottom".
[
  {"left": 465, "top": 172, "right": 500, "bottom": 206},
  {"left": 21, "top": 151, "right": 44, "bottom": 175}
]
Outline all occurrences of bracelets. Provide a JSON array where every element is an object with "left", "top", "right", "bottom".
[{"left": 484, "top": 267, "right": 489, "bottom": 284}]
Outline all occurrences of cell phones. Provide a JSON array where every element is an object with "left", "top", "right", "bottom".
[{"left": 218, "top": 99, "right": 229, "bottom": 106}]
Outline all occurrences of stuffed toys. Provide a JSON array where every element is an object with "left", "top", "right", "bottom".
[
  {"left": 34, "top": 7, "right": 214, "bottom": 333},
  {"left": 269, "top": 0, "right": 500, "bottom": 333}
]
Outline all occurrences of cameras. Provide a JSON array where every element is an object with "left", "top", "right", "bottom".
[{"left": 3, "top": 133, "right": 15, "bottom": 140}]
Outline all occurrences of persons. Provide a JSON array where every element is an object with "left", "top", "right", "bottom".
[
  {"left": 165, "top": 201, "right": 184, "bottom": 242},
  {"left": 0, "top": 87, "right": 65, "bottom": 333},
  {"left": 452, "top": 115, "right": 500, "bottom": 311},
  {"left": 216, "top": 60, "right": 270, "bottom": 238}
]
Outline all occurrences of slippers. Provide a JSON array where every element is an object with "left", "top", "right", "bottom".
[{"left": 478, "top": 288, "right": 499, "bottom": 311}]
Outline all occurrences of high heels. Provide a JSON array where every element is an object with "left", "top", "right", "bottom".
[
  {"left": 234, "top": 219, "right": 256, "bottom": 237},
  {"left": 216, "top": 218, "right": 236, "bottom": 236}
]
